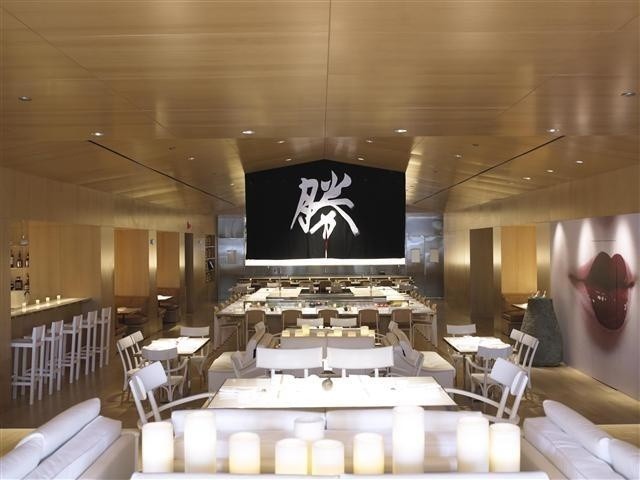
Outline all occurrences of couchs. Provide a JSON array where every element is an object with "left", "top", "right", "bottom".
[
  {"left": 520, "top": 416, "right": 627, "bottom": 480},
  {"left": 0, "top": 413, "right": 136, "bottom": 479}
]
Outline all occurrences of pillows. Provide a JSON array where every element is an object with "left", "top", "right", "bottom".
[
  {"left": 0, "top": 438, "right": 43, "bottom": 478},
  {"left": 543, "top": 400, "right": 612, "bottom": 466},
  {"left": 610, "top": 439, "right": 639, "bottom": 478},
  {"left": 14, "top": 398, "right": 101, "bottom": 459}
]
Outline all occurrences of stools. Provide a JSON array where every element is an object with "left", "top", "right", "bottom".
[
  {"left": 83, "top": 306, "right": 112, "bottom": 367},
  {"left": 10, "top": 325, "right": 46, "bottom": 405},
  {"left": 63, "top": 310, "right": 98, "bottom": 375},
  {"left": 46, "top": 314, "right": 83, "bottom": 383},
  {"left": 23, "top": 320, "right": 64, "bottom": 395}
]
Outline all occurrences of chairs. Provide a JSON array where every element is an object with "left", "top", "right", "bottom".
[
  {"left": 127, "top": 359, "right": 215, "bottom": 425},
  {"left": 446, "top": 357, "right": 530, "bottom": 426},
  {"left": 115, "top": 280, "right": 539, "bottom": 407}
]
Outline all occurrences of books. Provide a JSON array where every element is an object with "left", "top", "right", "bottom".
[{"left": 204, "top": 234, "right": 215, "bottom": 281}]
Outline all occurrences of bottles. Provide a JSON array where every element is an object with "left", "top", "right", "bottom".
[{"left": 9, "top": 249, "right": 28, "bottom": 290}]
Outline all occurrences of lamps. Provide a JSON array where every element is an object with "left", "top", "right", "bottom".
[
  {"left": 351, "top": 431, "right": 386, "bottom": 473},
  {"left": 455, "top": 409, "right": 489, "bottom": 477},
  {"left": 228, "top": 432, "right": 262, "bottom": 474},
  {"left": 392, "top": 404, "right": 425, "bottom": 474},
  {"left": 275, "top": 439, "right": 308, "bottom": 474},
  {"left": 293, "top": 415, "right": 325, "bottom": 440},
  {"left": 311, "top": 440, "right": 346, "bottom": 474},
  {"left": 489, "top": 422, "right": 521, "bottom": 476},
  {"left": 183, "top": 417, "right": 217, "bottom": 475},
  {"left": 141, "top": 421, "right": 175, "bottom": 475}
]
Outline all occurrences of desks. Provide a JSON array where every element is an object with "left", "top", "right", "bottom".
[{"left": 11, "top": 297, "right": 90, "bottom": 320}]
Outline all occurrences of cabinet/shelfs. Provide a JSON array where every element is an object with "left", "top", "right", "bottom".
[
  {"left": 204, "top": 233, "right": 216, "bottom": 282},
  {"left": 9, "top": 245, "right": 29, "bottom": 292},
  {"left": 217, "top": 213, "right": 245, "bottom": 302}
]
